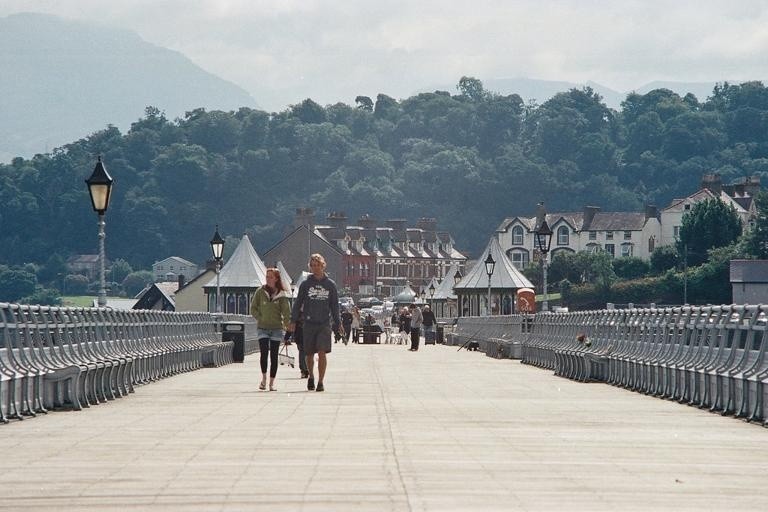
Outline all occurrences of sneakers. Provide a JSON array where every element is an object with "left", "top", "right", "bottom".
[
  {"left": 307, "top": 378, "right": 315, "bottom": 390},
  {"left": 335, "top": 337, "right": 408, "bottom": 345},
  {"left": 316, "top": 383, "right": 324, "bottom": 391}
]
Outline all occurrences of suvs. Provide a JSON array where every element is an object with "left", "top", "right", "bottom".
[{"left": 338, "top": 296, "right": 355, "bottom": 313}]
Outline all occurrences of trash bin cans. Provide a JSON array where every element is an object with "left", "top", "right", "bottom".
[
  {"left": 363, "top": 326, "right": 377, "bottom": 344},
  {"left": 222, "top": 321, "right": 245, "bottom": 363},
  {"left": 436, "top": 322, "right": 453, "bottom": 344}
]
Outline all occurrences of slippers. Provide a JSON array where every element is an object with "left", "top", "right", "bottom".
[
  {"left": 269, "top": 384, "right": 277, "bottom": 391},
  {"left": 259, "top": 381, "right": 266, "bottom": 390}
]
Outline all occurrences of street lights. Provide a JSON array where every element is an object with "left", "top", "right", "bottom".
[
  {"left": 210, "top": 223, "right": 225, "bottom": 313},
  {"left": 453, "top": 270, "right": 464, "bottom": 317},
  {"left": 536, "top": 213, "right": 553, "bottom": 313},
  {"left": 413, "top": 292, "right": 419, "bottom": 302},
  {"left": 420, "top": 289, "right": 427, "bottom": 303},
  {"left": 482, "top": 247, "right": 496, "bottom": 316},
  {"left": 428, "top": 283, "right": 436, "bottom": 316},
  {"left": 289, "top": 280, "right": 295, "bottom": 309},
  {"left": 85, "top": 154, "right": 114, "bottom": 307}
]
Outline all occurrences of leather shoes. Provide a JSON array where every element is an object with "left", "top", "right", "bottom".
[
  {"left": 301, "top": 373, "right": 308, "bottom": 378},
  {"left": 411, "top": 348, "right": 417, "bottom": 351}
]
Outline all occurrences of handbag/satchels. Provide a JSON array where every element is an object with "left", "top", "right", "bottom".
[{"left": 279, "top": 344, "right": 295, "bottom": 369}]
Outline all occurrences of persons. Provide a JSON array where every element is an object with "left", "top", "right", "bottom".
[
  {"left": 331, "top": 303, "right": 437, "bottom": 352},
  {"left": 285, "top": 302, "right": 309, "bottom": 378},
  {"left": 251, "top": 268, "right": 292, "bottom": 391},
  {"left": 288, "top": 253, "right": 345, "bottom": 392}
]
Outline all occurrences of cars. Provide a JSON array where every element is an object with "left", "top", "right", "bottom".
[
  {"left": 357, "top": 297, "right": 372, "bottom": 308},
  {"left": 370, "top": 296, "right": 383, "bottom": 305}
]
